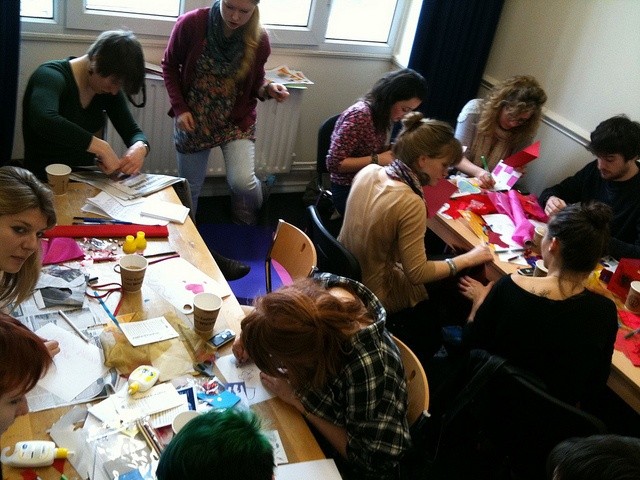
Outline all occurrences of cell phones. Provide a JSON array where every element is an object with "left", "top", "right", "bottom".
[
  {"left": 519, "top": 267, "right": 535, "bottom": 277},
  {"left": 513, "top": 183, "right": 530, "bottom": 195}
]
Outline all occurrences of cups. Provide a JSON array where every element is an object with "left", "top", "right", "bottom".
[
  {"left": 532, "top": 259, "right": 549, "bottom": 278},
  {"left": 193, "top": 293, "right": 222, "bottom": 333},
  {"left": 171, "top": 410, "right": 201, "bottom": 438},
  {"left": 531, "top": 227, "right": 545, "bottom": 255},
  {"left": 623, "top": 281, "right": 640, "bottom": 316},
  {"left": 114, "top": 253, "right": 148, "bottom": 291},
  {"left": 45, "top": 163, "right": 72, "bottom": 196}
]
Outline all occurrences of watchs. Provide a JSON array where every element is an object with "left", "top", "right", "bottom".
[{"left": 138, "top": 138, "right": 152, "bottom": 160}]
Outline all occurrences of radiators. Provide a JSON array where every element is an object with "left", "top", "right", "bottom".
[{"left": 102, "top": 79, "right": 303, "bottom": 179}]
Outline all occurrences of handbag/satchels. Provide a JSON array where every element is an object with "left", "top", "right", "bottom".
[{"left": 287, "top": 182, "right": 335, "bottom": 230}]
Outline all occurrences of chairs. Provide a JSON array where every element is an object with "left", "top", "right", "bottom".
[
  {"left": 390, "top": 333, "right": 430, "bottom": 436},
  {"left": 461, "top": 348, "right": 608, "bottom": 480},
  {"left": 300, "top": 113, "right": 345, "bottom": 232},
  {"left": 303, "top": 205, "right": 363, "bottom": 281},
  {"left": 266, "top": 219, "right": 317, "bottom": 294}
]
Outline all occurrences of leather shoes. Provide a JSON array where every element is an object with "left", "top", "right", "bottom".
[{"left": 206, "top": 248, "right": 250, "bottom": 281}]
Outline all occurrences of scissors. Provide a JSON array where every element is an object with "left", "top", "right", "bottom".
[{"left": 193, "top": 359, "right": 225, "bottom": 388}]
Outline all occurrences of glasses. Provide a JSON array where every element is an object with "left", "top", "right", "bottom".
[{"left": 506, "top": 106, "right": 529, "bottom": 126}]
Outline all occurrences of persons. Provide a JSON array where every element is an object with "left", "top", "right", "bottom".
[
  {"left": 152, "top": 405, "right": 278, "bottom": 480},
  {"left": 158, "top": 0, "right": 289, "bottom": 226},
  {"left": 0, "top": 306, "right": 55, "bottom": 439},
  {"left": 325, "top": 65, "right": 428, "bottom": 219},
  {"left": 448, "top": 73, "right": 549, "bottom": 192},
  {"left": 229, "top": 269, "right": 414, "bottom": 480},
  {"left": 21, "top": 26, "right": 151, "bottom": 185},
  {"left": 336, "top": 110, "right": 494, "bottom": 361},
  {"left": 451, "top": 195, "right": 618, "bottom": 416},
  {"left": 548, "top": 431, "right": 639, "bottom": 480},
  {"left": 535, "top": 111, "right": 639, "bottom": 261},
  {"left": 0, "top": 162, "right": 56, "bottom": 316}
]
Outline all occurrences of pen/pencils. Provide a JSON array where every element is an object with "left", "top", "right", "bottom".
[
  {"left": 619, "top": 323, "right": 639, "bottom": 336},
  {"left": 138, "top": 419, "right": 161, "bottom": 457},
  {"left": 58, "top": 309, "right": 90, "bottom": 343},
  {"left": 72, "top": 221, "right": 110, "bottom": 225},
  {"left": 136, "top": 420, "right": 159, "bottom": 457},
  {"left": 73, "top": 217, "right": 134, "bottom": 223},
  {"left": 508, "top": 251, "right": 532, "bottom": 261},
  {"left": 492, "top": 249, "right": 526, "bottom": 253},
  {"left": 148, "top": 255, "right": 180, "bottom": 265},
  {"left": 481, "top": 156, "right": 492, "bottom": 180},
  {"left": 142, "top": 251, "right": 178, "bottom": 258},
  {"left": 144, "top": 423, "right": 164, "bottom": 453},
  {"left": 624, "top": 327, "right": 640, "bottom": 340}
]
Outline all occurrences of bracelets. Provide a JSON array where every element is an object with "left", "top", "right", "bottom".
[
  {"left": 365, "top": 151, "right": 386, "bottom": 169},
  {"left": 435, "top": 251, "right": 459, "bottom": 279}
]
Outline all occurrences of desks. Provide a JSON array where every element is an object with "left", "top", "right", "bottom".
[
  {"left": 0, "top": 176, "right": 341, "bottom": 479},
  {"left": 422, "top": 180, "right": 639, "bottom": 413}
]
